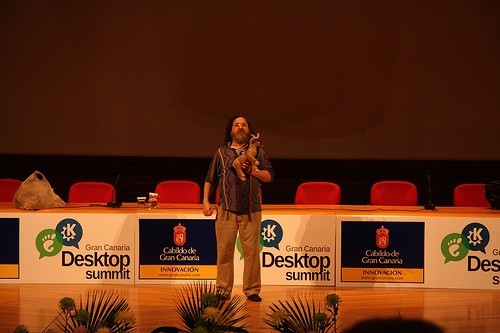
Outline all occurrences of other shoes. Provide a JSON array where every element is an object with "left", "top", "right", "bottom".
[
  {"left": 214, "top": 293, "right": 227, "bottom": 300},
  {"left": 249, "top": 294, "right": 262, "bottom": 302}
]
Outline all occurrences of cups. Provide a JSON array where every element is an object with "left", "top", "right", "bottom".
[{"left": 137, "top": 197, "right": 146, "bottom": 208}]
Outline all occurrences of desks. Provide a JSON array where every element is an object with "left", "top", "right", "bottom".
[{"left": 0, "top": 205, "right": 500, "bottom": 288}]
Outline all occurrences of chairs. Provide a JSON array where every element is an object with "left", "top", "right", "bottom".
[
  {"left": 296, "top": 182, "right": 342, "bottom": 204},
  {"left": 0, "top": 178, "right": 23, "bottom": 205},
  {"left": 68, "top": 182, "right": 117, "bottom": 205},
  {"left": 155, "top": 181, "right": 200, "bottom": 205},
  {"left": 369, "top": 181, "right": 418, "bottom": 206},
  {"left": 454, "top": 182, "right": 492, "bottom": 207}
]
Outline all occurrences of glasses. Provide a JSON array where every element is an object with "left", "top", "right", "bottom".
[{"left": 233, "top": 123, "right": 249, "bottom": 128}]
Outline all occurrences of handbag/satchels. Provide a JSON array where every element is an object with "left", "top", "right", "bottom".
[{"left": 13, "top": 171, "right": 67, "bottom": 211}]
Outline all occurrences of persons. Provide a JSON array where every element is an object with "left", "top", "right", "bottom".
[{"left": 200, "top": 113, "right": 275, "bottom": 302}]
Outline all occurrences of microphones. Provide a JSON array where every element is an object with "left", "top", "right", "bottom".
[
  {"left": 107, "top": 175, "right": 122, "bottom": 207},
  {"left": 424, "top": 174, "right": 435, "bottom": 210}
]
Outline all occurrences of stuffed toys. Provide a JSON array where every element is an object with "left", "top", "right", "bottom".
[{"left": 232, "top": 133, "right": 263, "bottom": 183}]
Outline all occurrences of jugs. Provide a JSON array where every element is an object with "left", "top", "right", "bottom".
[{"left": 149, "top": 193, "right": 161, "bottom": 209}]
